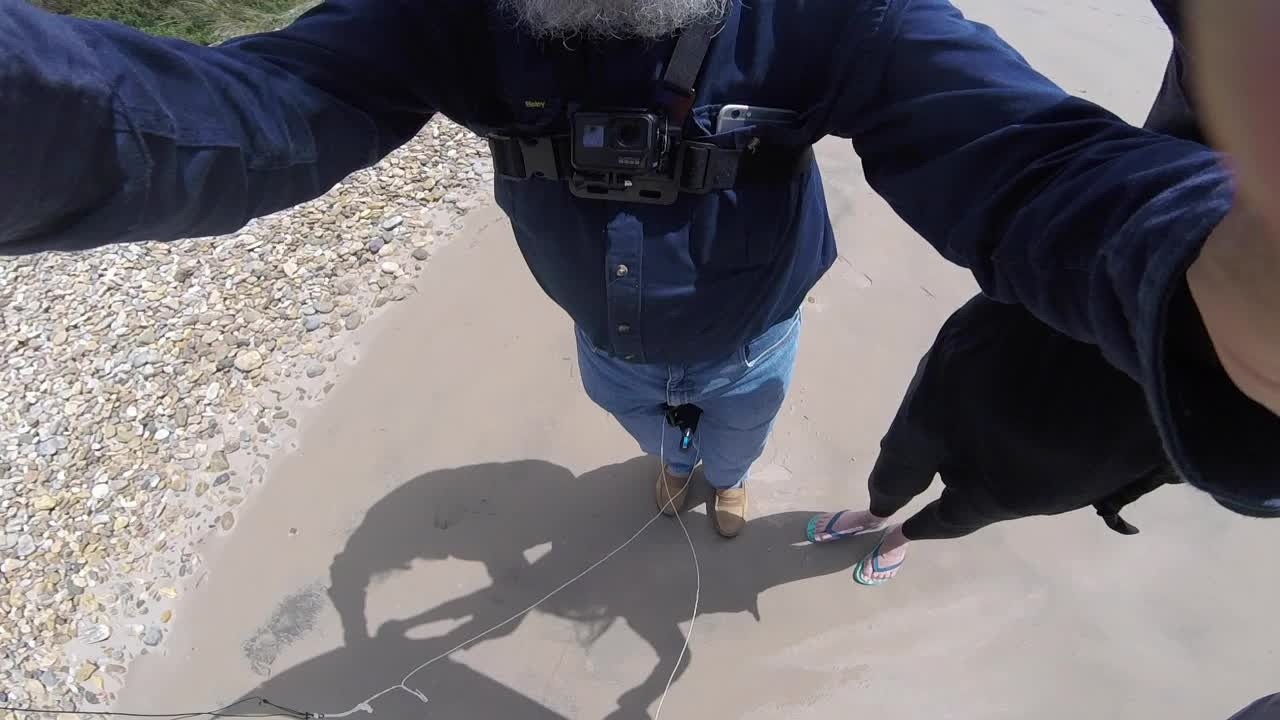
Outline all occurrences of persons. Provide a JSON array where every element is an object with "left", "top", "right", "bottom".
[
  {"left": 805, "top": 0, "right": 1280, "bottom": 586},
  {"left": 1, "top": 1, "right": 1280, "bottom": 537}
]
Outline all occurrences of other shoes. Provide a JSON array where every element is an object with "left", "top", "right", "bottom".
[
  {"left": 712, "top": 479, "right": 748, "bottom": 536},
  {"left": 655, "top": 463, "right": 691, "bottom": 516}
]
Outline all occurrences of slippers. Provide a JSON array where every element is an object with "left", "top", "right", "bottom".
[
  {"left": 805, "top": 509, "right": 859, "bottom": 541},
  {"left": 853, "top": 527, "right": 905, "bottom": 585}
]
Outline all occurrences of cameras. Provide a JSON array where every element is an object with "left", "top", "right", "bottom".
[{"left": 570, "top": 112, "right": 660, "bottom": 176}]
